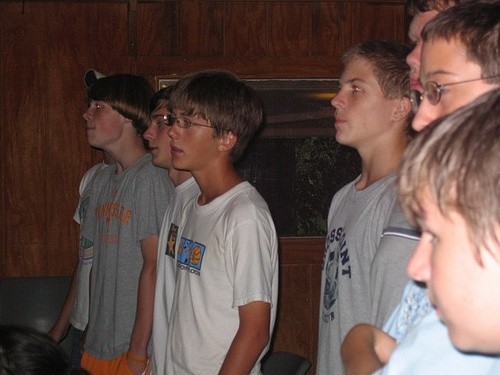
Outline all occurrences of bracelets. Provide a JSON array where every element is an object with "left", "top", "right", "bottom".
[{"left": 128, "top": 355, "right": 148, "bottom": 364}]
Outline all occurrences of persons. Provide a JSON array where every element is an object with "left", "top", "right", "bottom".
[
  {"left": 146, "top": 87, "right": 193, "bottom": 184},
  {"left": 0, "top": 325, "right": 92, "bottom": 375},
  {"left": 58, "top": 73, "right": 174, "bottom": 375},
  {"left": 47, "top": 74, "right": 113, "bottom": 375},
  {"left": 152, "top": 71, "right": 279, "bottom": 375},
  {"left": 315, "top": 1, "right": 500, "bottom": 375},
  {"left": 397, "top": 90, "right": 499, "bottom": 353}
]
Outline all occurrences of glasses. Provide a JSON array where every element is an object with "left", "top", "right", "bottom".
[
  {"left": 163, "top": 113, "right": 231, "bottom": 132},
  {"left": 410, "top": 75, "right": 500, "bottom": 113}
]
